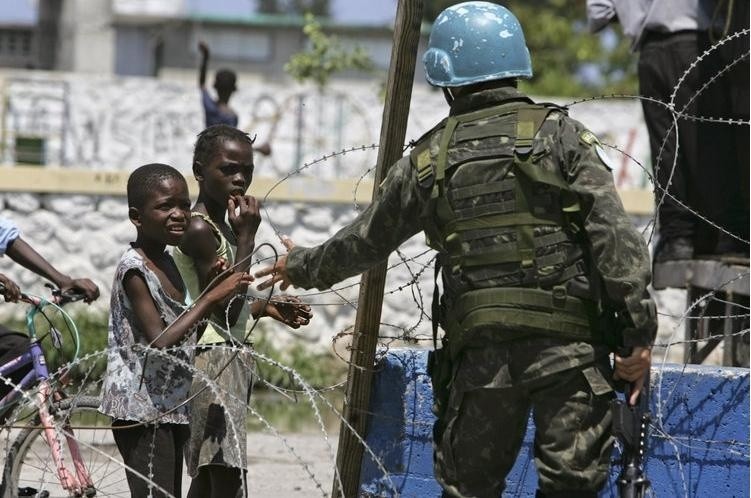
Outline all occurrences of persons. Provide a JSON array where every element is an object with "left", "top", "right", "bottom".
[
  {"left": 95, "top": 162, "right": 256, "bottom": 498},
  {"left": 167, "top": 124, "right": 315, "bottom": 497},
  {"left": 0, "top": 213, "right": 101, "bottom": 498},
  {"left": 579, "top": 0, "right": 699, "bottom": 262},
  {"left": 253, "top": 0, "right": 659, "bottom": 498},
  {"left": 195, "top": 37, "right": 274, "bottom": 157}
]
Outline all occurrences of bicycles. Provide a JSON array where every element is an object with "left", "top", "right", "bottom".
[{"left": 1, "top": 280, "right": 132, "bottom": 498}]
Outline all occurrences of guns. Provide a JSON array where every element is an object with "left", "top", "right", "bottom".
[{"left": 611, "top": 336, "right": 652, "bottom": 497}]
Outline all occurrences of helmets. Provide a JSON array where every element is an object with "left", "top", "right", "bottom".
[{"left": 423, "top": 2, "right": 535, "bottom": 89}]
